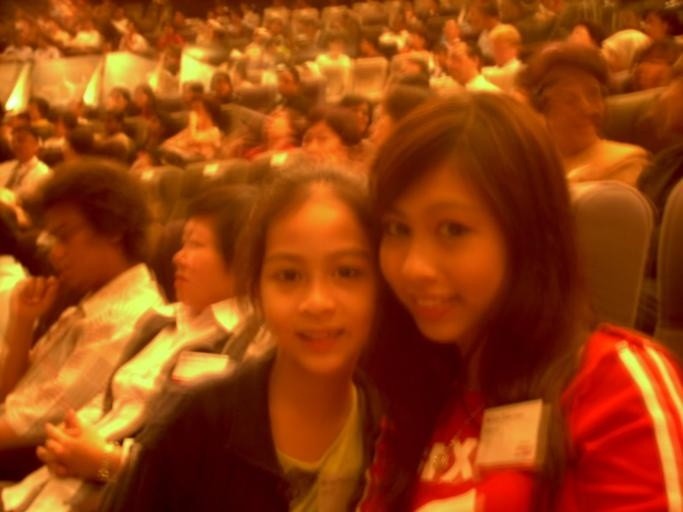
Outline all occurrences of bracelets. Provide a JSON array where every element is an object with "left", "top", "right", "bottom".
[{"left": 95, "top": 437, "right": 115, "bottom": 487}]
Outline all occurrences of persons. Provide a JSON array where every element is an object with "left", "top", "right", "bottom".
[
  {"left": 1, "top": 157, "right": 173, "bottom": 481},
  {"left": 0, "top": 1, "right": 682, "bottom": 341},
  {"left": 123, "top": 170, "right": 380, "bottom": 512},
  {"left": 0, "top": 185, "right": 279, "bottom": 512},
  {"left": 354, "top": 92, "right": 679, "bottom": 512}
]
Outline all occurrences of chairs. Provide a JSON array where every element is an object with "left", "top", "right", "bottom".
[
  {"left": 341, "top": 1, "right": 683, "bottom": 370},
  {"left": 0, "top": 0, "right": 341, "bottom": 303}
]
